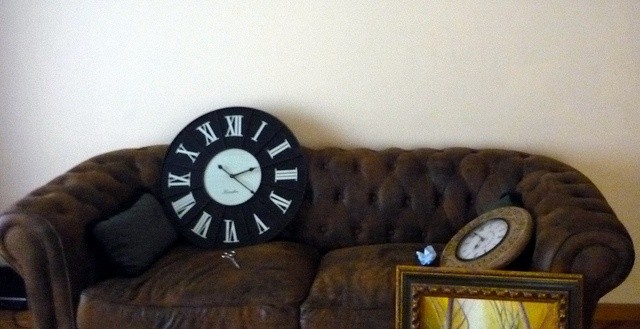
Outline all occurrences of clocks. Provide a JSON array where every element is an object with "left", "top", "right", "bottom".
[
  {"left": 439, "top": 205, "right": 534, "bottom": 271},
  {"left": 157, "top": 106, "right": 308, "bottom": 251}
]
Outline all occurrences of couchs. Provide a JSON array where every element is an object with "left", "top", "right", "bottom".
[{"left": 0, "top": 144, "right": 573, "bottom": 329}]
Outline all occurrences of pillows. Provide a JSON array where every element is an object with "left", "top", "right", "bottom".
[{"left": 92, "top": 193, "right": 178, "bottom": 278}]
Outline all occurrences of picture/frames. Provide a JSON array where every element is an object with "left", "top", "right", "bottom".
[{"left": 394, "top": 264, "right": 584, "bottom": 329}]
